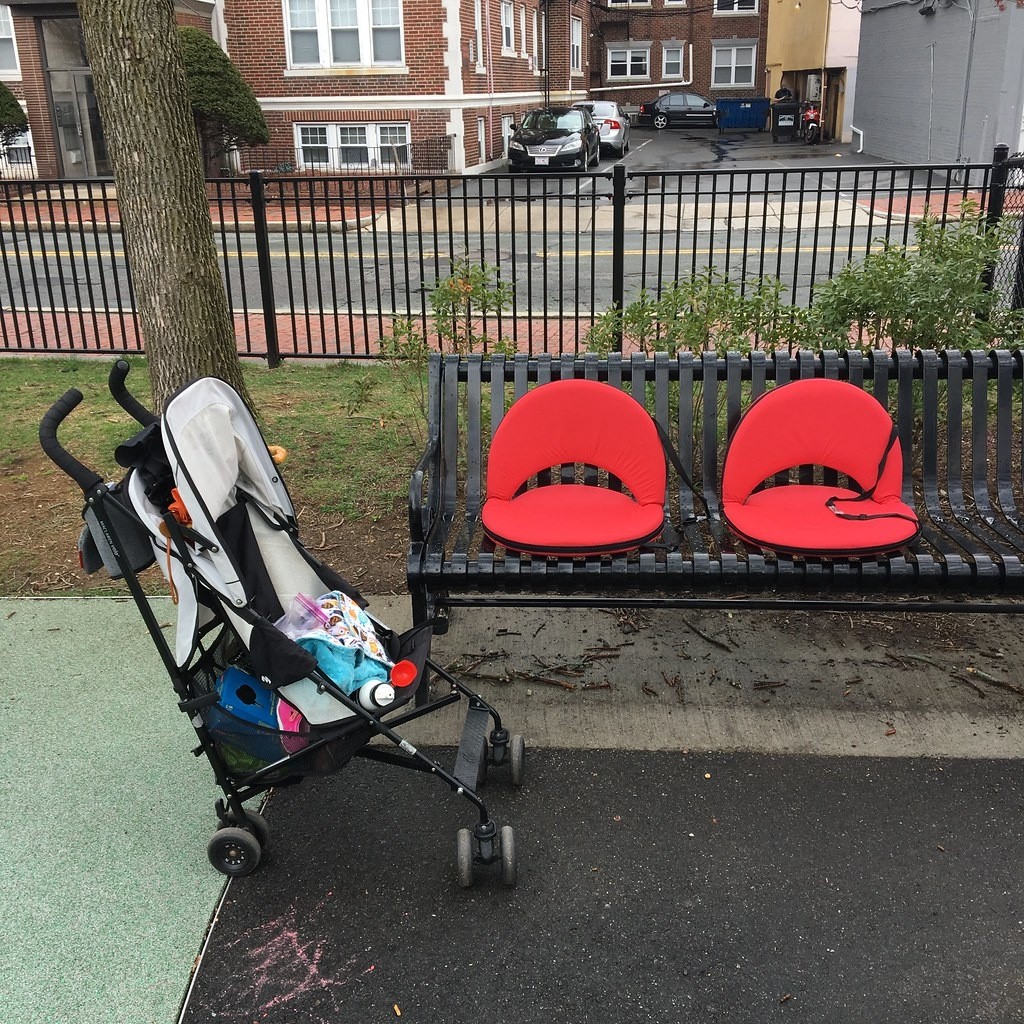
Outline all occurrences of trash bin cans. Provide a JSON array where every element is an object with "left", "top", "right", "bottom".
[
  {"left": 772, "top": 102, "right": 799, "bottom": 143},
  {"left": 715, "top": 96, "right": 771, "bottom": 135}
]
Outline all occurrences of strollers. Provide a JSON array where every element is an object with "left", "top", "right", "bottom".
[{"left": 37, "top": 361, "right": 529, "bottom": 890}]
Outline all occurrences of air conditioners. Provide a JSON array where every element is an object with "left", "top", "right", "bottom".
[{"left": 805, "top": 74, "right": 821, "bottom": 101}]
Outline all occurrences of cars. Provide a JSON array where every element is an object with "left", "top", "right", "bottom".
[
  {"left": 638, "top": 90, "right": 719, "bottom": 129},
  {"left": 507, "top": 106, "right": 602, "bottom": 172},
  {"left": 571, "top": 99, "right": 631, "bottom": 157}
]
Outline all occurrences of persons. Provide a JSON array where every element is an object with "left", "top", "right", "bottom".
[{"left": 774, "top": 80, "right": 792, "bottom": 103}]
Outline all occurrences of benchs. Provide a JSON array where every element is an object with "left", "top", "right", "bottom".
[{"left": 403, "top": 350, "right": 1024, "bottom": 706}]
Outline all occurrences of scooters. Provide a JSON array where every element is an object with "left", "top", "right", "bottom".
[{"left": 795, "top": 99, "right": 821, "bottom": 143}]
